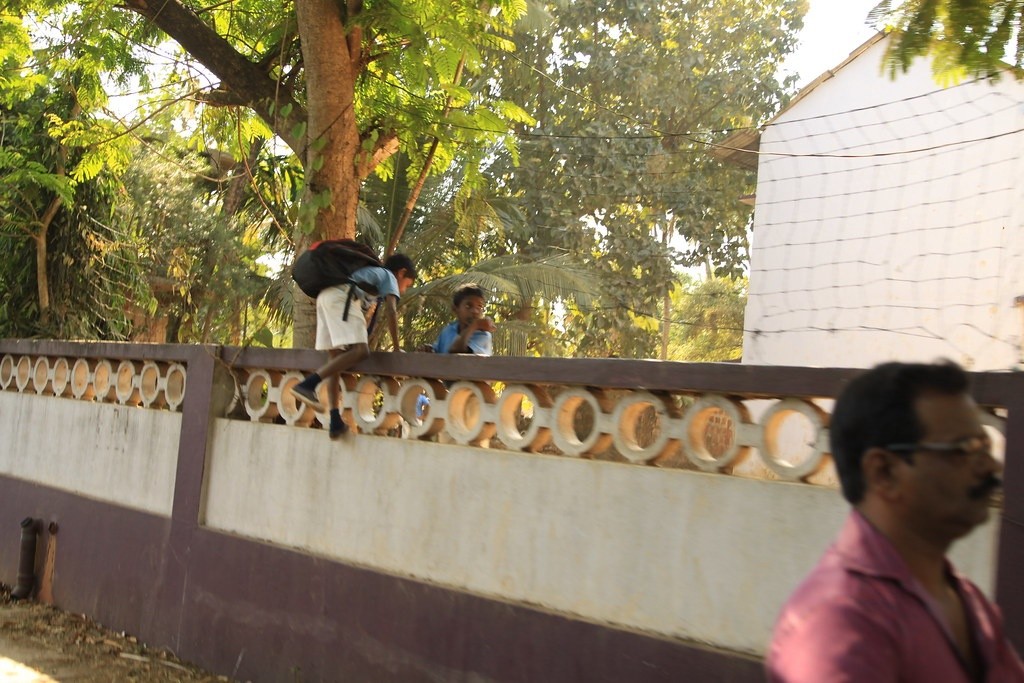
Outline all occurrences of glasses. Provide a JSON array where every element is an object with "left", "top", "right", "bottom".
[{"left": 886, "top": 435, "right": 991, "bottom": 456}]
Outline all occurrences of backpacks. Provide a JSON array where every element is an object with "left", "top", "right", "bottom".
[{"left": 291, "top": 239, "right": 388, "bottom": 298}]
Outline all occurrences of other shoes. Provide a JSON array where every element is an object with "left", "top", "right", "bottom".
[
  {"left": 330, "top": 424, "right": 349, "bottom": 438},
  {"left": 290, "top": 384, "right": 326, "bottom": 413}
]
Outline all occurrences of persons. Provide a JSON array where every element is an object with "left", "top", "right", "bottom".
[
  {"left": 288, "top": 254, "right": 418, "bottom": 441},
  {"left": 387, "top": 286, "right": 497, "bottom": 439},
  {"left": 761, "top": 355, "right": 1023, "bottom": 683}
]
